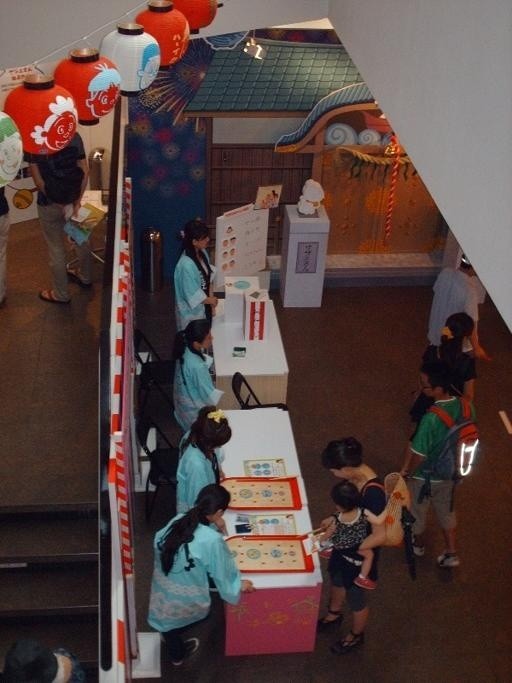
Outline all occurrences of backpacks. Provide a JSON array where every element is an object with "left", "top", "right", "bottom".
[{"left": 425, "top": 395, "right": 482, "bottom": 483}]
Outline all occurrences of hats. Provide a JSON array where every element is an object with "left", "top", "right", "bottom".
[{"left": 460, "top": 252, "right": 471, "bottom": 266}]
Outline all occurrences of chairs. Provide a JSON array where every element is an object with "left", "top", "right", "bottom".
[
  {"left": 133, "top": 326, "right": 174, "bottom": 411},
  {"left": 224, "top": 276, "right": 260, "bottom": 299},
  {"left": 135, "top": 419, "right": 177, "bottom": 522},
  {"left": 231, "top": 372, "right": 288, "bottom": 409}
]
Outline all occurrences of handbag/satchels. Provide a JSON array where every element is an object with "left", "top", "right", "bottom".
[
  {"left": 42, "top": 165, "right": 85, "bottom": 204},
  {"left": 362, "top": 471, "right": 415, "bottom": 549}
]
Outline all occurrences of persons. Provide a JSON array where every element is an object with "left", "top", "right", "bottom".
[
  {"left": 317, "top": 435, "right": 387, "bottom": 657},
  {"left": 176, "top": 405, "right": 231, "bottom": 537},
  {"left": 171, "top": 317, "right": 224, "bottom": 431},
  {"left": 1, "top": 186, "right": 11, "bottom": 308},
  {"left": 145, "top": 483, "right": 257, "bottom": 667},
  {"left": 172, "top": 215, "right": 219, "bottom": 345},
  {"left": 1, "top": 641, "right": 88, "bottom": 683},
  {"left": 319, "top": 478, "right": 388, "bottom": 591},
  {"left": 25, "top": 130, "right": 94, "bottom": 306},
  {"left": 399, "top": 251, "right": 488, "bottom": 569}
]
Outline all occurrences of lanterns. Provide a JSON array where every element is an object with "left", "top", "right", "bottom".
[
  {"left": 136, "top": 2, "right": 190, "bottom": 71},
  {"left": 54, "top": 48, "right": 121, "bottom": 127},
  {"left": 174, "top": 1, "right": 217, "bottom": 35},
  {"left": 100, "top": 23, "right": 161, "bottom": 97},
  {"left": 3, "top": 73, "right": 78, "bottom": 158},
  {"left": 1, "top": 107, "right": 23, "bottom": 186}
]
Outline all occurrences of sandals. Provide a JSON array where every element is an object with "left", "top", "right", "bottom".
[
  {"left": 316, "top": 608, "right": 344, "bottom": 631},
  {"left": 329, "top": 630, "right": 366, "bottom": 656}
]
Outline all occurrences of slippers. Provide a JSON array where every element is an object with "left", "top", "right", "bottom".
[
  {"left": 39, "top": 286, "right": 71, "bottom": 304},
  {"left": 66, "top": 264, "right": 92, "bottom": 288}
]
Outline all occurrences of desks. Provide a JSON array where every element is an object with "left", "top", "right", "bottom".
[
  {"left": 209, "top": 409, "right": 325, "bottom": 658},
  {"left": 206, "top": 298, "right": 289, "bottom": 409}
]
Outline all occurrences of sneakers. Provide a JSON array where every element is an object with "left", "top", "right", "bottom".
[
  {"left": 171, "top": 636, "right": 200, "bottom": 667},
  {"left": 412, "top": 536, "right": 425, "bottom": 557},
  {"left": 435, "top": 550, "right": 460, "bottom": 568},
  {"left": 351, "top": 574, "right": 380, "bottom": 591}
]
